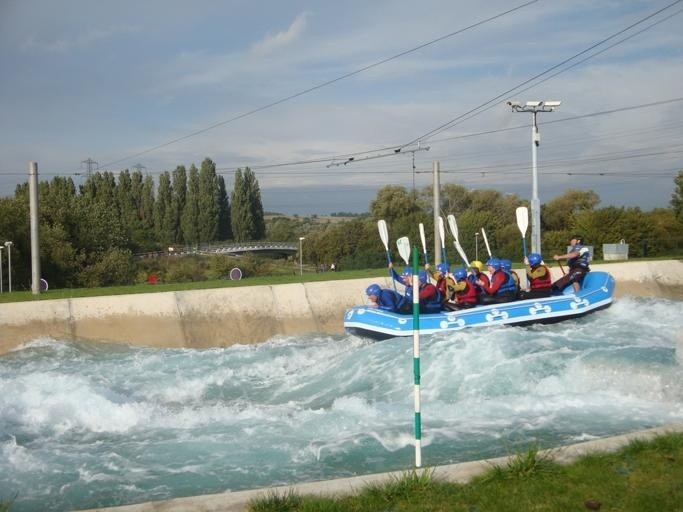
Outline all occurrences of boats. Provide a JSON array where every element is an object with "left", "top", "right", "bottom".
[{"left": 344, "top": 272, "right": 615, "bottom": 341}]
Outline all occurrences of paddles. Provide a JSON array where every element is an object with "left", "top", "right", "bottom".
[
  {"left": 516, "top": 206, "right": 529, "bottom": 287},
  {"left": 482, "top": 226, "right": 492, "bottom": 259},
  {"left": 396, "top": 236, "right": 411, "bottom": 266},
  {"left": 436, "top": 216, "right": 448, "bottom": 276},
  {"left": 418, "top": 223, "right": 430, "bottom": 283},
  {"left": 453, "top": 241, "right": 486, "bottom": 295},
  {"left": 377, "top": 219, "right": 396, "bottom": 292},
  {"left": 448, "top": 214, "right": 466, "bottom": 270}
]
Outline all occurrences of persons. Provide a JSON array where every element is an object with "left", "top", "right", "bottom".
[{"left": 366, "top": 234, "right": 590, "bottom": 314}]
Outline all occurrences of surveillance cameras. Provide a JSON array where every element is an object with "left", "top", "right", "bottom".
[
  {"left": 544, "top": 100, "right": 563, "bottom": 108},
  {"left": 526, "top": 100, "right": 543, "bottom": 107},
  {"left": 507, "top": 100, "right": 523, "bottom": 108}
]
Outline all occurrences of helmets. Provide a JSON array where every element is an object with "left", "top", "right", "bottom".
[
  {"left": 366, "top": 284, "right": 381, "bottom": 297},
  {"left": 486, "top": 259, "right": 511, "bottom": 270},
  {"left": 454, "top": 268, "right": 467, "bottom": 283},
  {"left": 528, "top": 253, "right": 542, "bottom": 267},
  {"left": 403, "top": 267, "right": 412, "bottom": 276},
  {"left": 569, "top": 235, "right": 583, "bottom": 240},
  {"left": 419, "top": 271, "right": 427, "bottom": 283},
  {"left": 469, "top": 261, "right": 482, "bottom": 272},
  {"left": 438, "top": 263, "right": 449, "bottom": 272}
]
